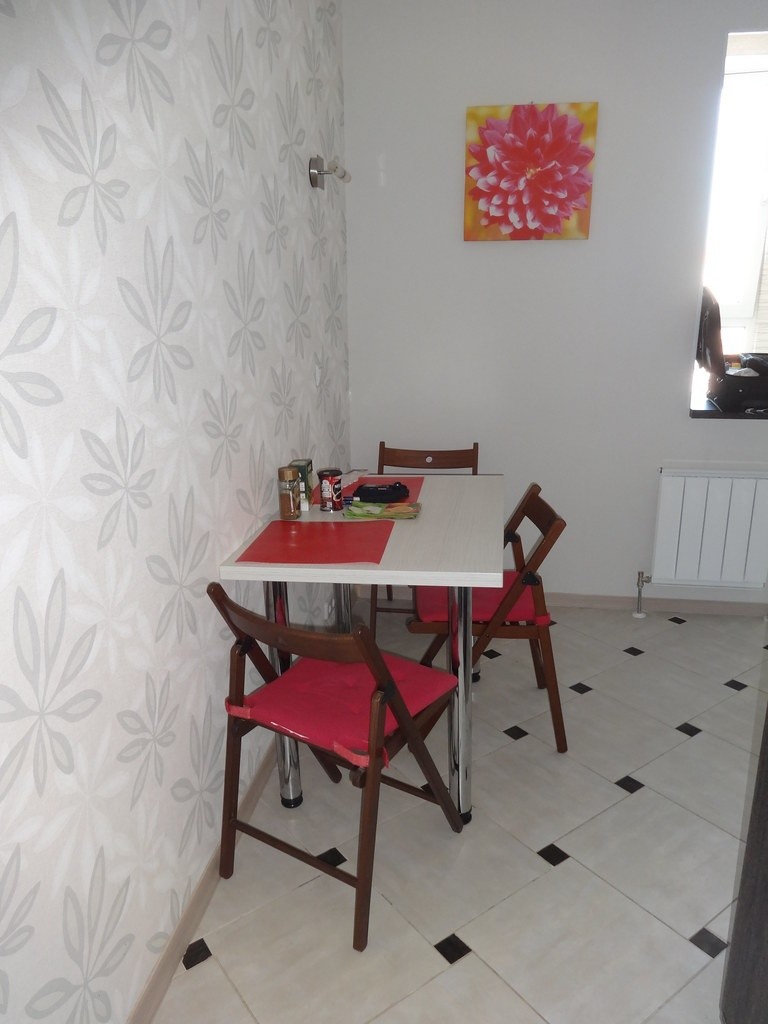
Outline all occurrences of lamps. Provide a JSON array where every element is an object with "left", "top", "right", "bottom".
[{"left": 309, "top": 156, "right": 352, "bottom": 189}]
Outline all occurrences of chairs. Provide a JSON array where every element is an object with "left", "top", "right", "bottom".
[
  {"left": 406, "top": 482, "right": 568, "bottom": 754},
  {"left": 369, "top": 441, "right": 479, "bottom": 647},
  {"left": 206, "top": 580, "right": 463, "bottom": 952}
]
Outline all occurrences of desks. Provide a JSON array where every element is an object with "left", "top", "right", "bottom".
[{"left": 219, "top": 475, "right": 504, "bottom": 589}]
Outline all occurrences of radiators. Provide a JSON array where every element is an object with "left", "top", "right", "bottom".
[{"left": 631, "top": 468, "right": 768, "bottom": 619}]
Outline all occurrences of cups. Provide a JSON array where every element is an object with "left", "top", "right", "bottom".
[
  {"left": 317, "top": 470, "right": 342, "bottom": 511},
  {"left": 277, "top": 467, "right": 302, "bottom": 519}
]
